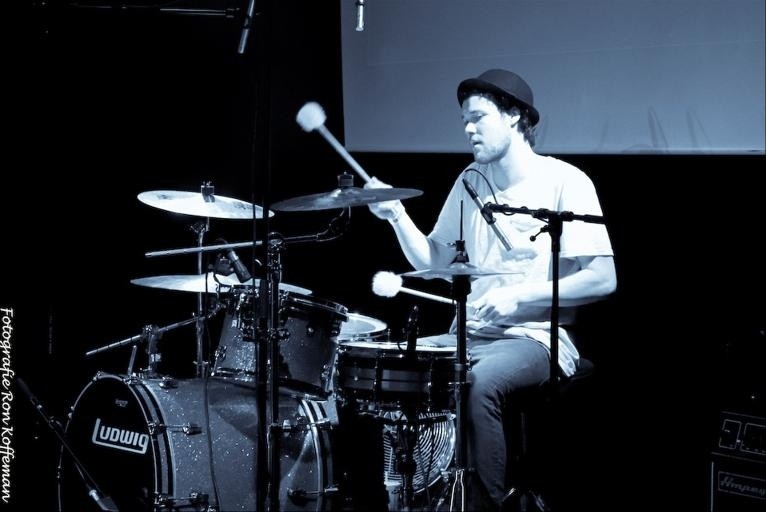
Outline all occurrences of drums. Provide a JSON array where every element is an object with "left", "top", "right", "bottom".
[
  {"left": 336, "top": 314, "right": 389, "bottom": 343},
  {"left": 59, "top": 371, "right": 338, "bottom": 510},
  {"left": 334, "top": 340, "right": 471, "bottom": 410},
  {"left": 210, "top": 285, "right": 349, "bottom": 399}
]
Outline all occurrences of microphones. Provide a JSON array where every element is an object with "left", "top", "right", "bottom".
[
  {"left": 84, "top": 475, "right": 119, "bottom": 512},
  {"left": 462, "top": 179, "right": 514, "bottom": 252},
  {"left": 237, "top": 0, "right": 255, "bottom": 53},
  {"left": 218, "top": 240, "right": 251, "bottom": 283},
  {"left": 355, "top": 0, "right": 364, "bottom": 32}
]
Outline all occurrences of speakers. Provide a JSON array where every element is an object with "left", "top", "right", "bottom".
[{"left": 709, "top": 452, "right": 765, "bottom": 512}]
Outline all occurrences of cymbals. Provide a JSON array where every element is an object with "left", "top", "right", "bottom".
[
  {"left": 272, "top": 188, "right": 423, "bottom": 210},
  {"left": 130, "top": 272, "right": 313, "bottom": 297},
  {"left": 138, "top": 190, "right": 275, "bottom": 220},
  {"left": 397, "top": 263, "right": 525, "bottom": 277}
]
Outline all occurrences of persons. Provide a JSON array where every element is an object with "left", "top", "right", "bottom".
[{"left": 364, "top": 69, "right": 617, "bottom": 505}]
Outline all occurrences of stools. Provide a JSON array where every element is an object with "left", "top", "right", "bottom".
[{"left": 500, "top": 357, "right": 594, "bottom": 512}]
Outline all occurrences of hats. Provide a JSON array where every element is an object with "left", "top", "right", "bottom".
[{"left": 457, "top": 68, "right": 540, "bottom": 127}]
